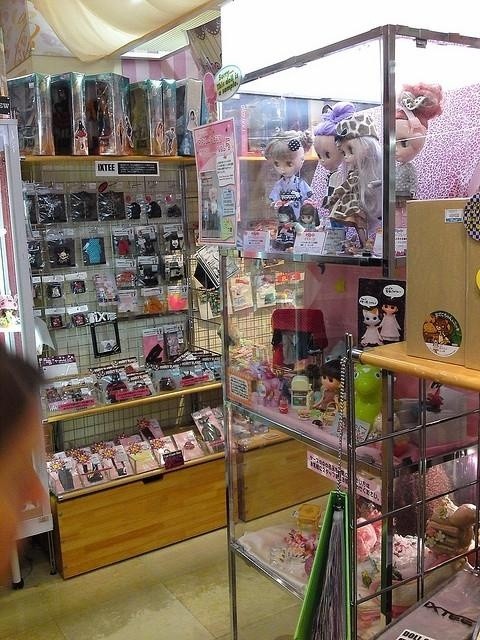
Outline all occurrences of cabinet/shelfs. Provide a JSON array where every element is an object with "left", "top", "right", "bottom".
[
  {"left": 16, "top": 154, "right": 239, "bottom": 577},
  {"left": 207, "top": 24, "right": 479, "bottom": 635},
  {"left": 339, "top": 331, "right": 479, "bottom": 636},
  {"left": 0, "top": 119, "right": 57, "bottom": 587}
]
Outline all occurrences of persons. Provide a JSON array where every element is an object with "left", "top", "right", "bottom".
[
  {"left": 75, "top": 121, "right": 86, "bottom": 150},
  {"left": 156, "top": 124, "right": 164, "bottom": 150},
  {"left": 395, "top": 84, "right": 445, "bottom": 255},
  {"left": 0, "top": 348, "right": 46, "bottom": 592},
  {"left": 298, "top": 203, "right": 320, "bottom": 230},
  {"left": 268, "top": 204, "right": 304, "bottom": 252},
  {"left": 187, "top": 110, "right": 197, "bottom": 130},
  {"left": 166, "top": 128, "right": 176, "bottom": 153},
  {"left": 336, "top": 115, "right": 383, "bottom": 256},
  {"left": 126, "top": 116, "right": 133, "bottom": 147},
  {"left": 310, "top": 360, "right": 342, "bottom": 411},
  {"left": 266, "top": 131, "right": 320, "bottom": 253},
  {"left": 87, "top": 83, "right": 111, "bottom": 154},
  {"left": 54, "top": 87, "right": 71, "bottom": 148},
  {"left": 312, "top": 101, "right": 362, "bottom": 252},
  {"left": 116, "top": 119, "right": 124, "bottom": 145}
]
